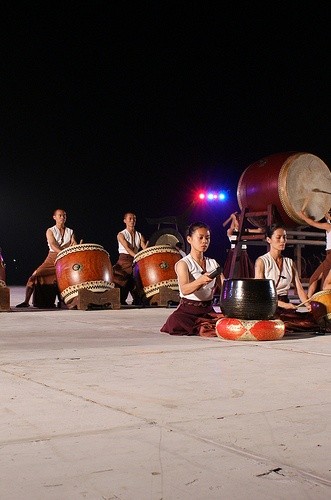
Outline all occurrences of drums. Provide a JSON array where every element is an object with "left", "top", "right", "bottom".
[
  {"left": 309, "top": 290, "right": 331, "bottom": 321},
  {"left": 54, "top": 243, "right": 115, "bottom": 304},
  {"left": 236, "top": 150, "right": 330, "bottom": 230},
  {"left": 131, "top": 244, "right": 185, "bottom": 305},
  {"left": 1, "top": 254, "right": 7, "bottom": 288}
]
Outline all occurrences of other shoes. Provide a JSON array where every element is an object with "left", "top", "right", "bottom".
[{"left": 16, "top": 303, "right": 28, "bottom": 308}]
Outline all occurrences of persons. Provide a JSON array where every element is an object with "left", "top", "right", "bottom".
[
  {"left": 15, "top": 209, "right": 78, "bottom": 308},
  {"left": 113, "top": 210, "right": 150, "bottom": 305},
  {"left": 212, "top": 213, "right": 265, "bottom": 305},
  {"left": 255, "top": 223, "right": 331, "bottom": 336},
  {"left": 299, "top": 208, "right": 331, "bottom": 307},
  {"left": 160, "top": 221, "right": 226, "bottom": 338},
  {"left": 175, "top": 242, "right": 186, "bottom": 258}
]
